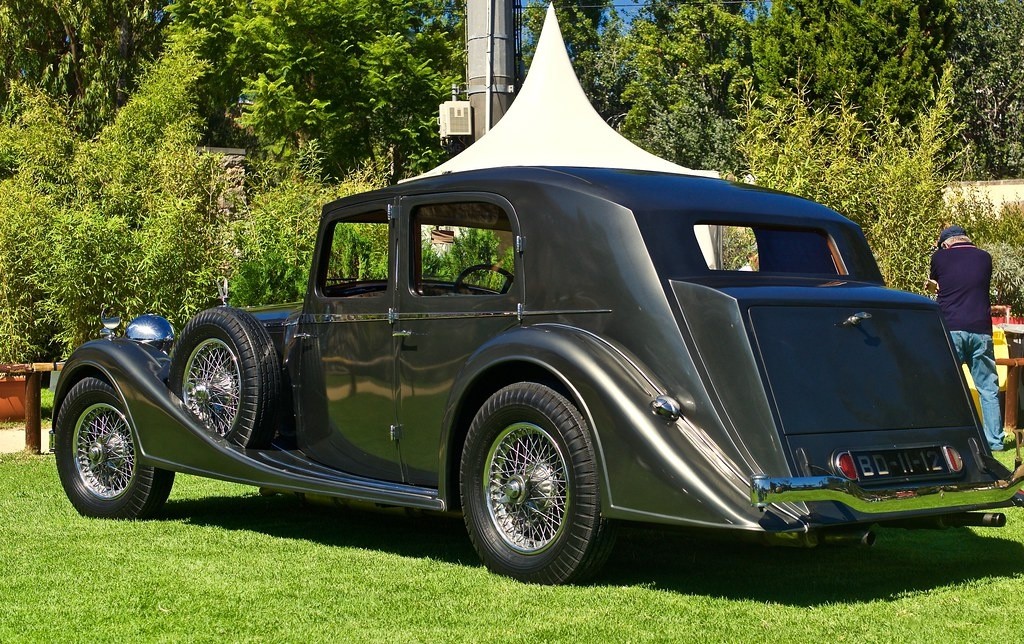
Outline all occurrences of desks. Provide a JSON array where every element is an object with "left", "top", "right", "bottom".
[
  {"left": 995, "top": 358, "right": 1024, "bottom": 432},
  {"left": 0, "top": 362, "right": 67, "bottom": 455}
]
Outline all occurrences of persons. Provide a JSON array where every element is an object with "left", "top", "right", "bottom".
[{"left": 924, "top": 225, "right": 1005, "bottom": 451}]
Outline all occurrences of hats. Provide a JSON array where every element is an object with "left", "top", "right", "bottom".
[{"left": 938, "top": 226, "right": 965, "bottom": 249}]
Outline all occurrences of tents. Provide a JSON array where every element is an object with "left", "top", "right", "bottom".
[{"left": 396, "top": 0, "right": 723, "bottom": 271}]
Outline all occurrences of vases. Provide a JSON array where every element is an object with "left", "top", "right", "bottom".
[{"left": 0, "top": 378, "right": 26, "bottom": 422}]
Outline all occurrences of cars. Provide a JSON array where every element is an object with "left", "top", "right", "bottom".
[{"left": 50, "top": 164, "right": 1024, "bottom": 591}]
